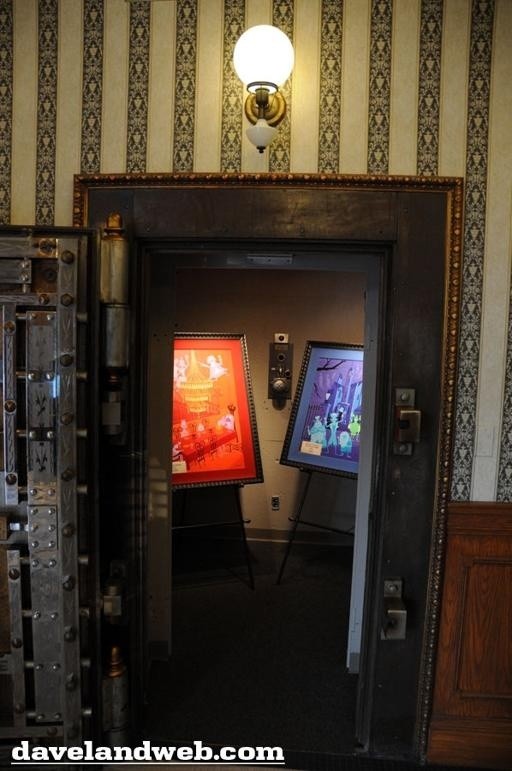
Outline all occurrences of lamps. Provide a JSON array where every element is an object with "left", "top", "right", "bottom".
[{"left": 229, "top": 25, "right": 297, "bottom": 156}]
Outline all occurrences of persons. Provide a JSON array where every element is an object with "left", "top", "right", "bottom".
[{"left": 306, "top": 413, "right": 361, "bottom": 462}]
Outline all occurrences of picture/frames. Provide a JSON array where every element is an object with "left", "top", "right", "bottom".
[
  {"left": 280, "top": 336, "right": 364, "bottom": 481},
  {"left": 173, "top": 330, "right": 263, "bottom": 490}
]
